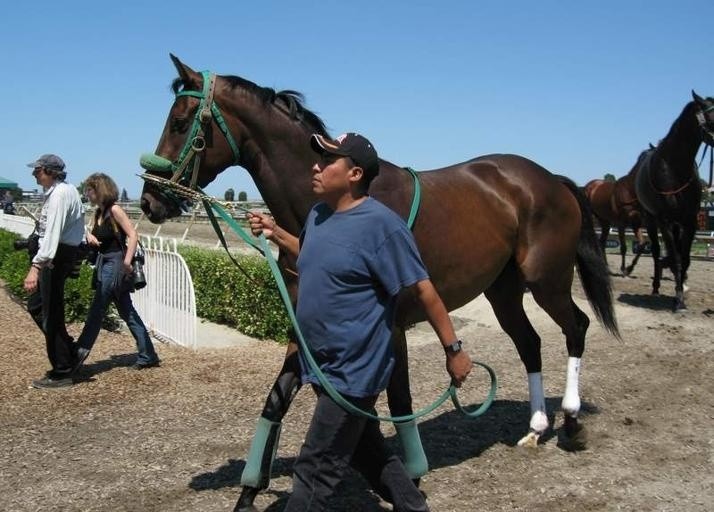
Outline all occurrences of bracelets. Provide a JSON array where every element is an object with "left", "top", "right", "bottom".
[{"left": 31, "top": 265, "right": 42, "bottom": 271}]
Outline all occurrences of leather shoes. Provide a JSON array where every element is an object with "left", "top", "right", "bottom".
[
  {"left": 32, "top": 345, "right": 91, "bottom": 389},
  {"left": 130, "top": 360, "right": 160, "bottom": 370}
]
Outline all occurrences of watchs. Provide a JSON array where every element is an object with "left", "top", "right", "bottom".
[{"left": 444, "top": 341, "right": 464, "bottom": 355}]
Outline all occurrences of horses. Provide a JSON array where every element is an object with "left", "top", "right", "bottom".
[
  {"left": 635, "top": 89, "right": 714, "bottom": 315},
  {"left": 138, "top": 53, "right": 624, "bottom": 512},
  {"left": 581, "top": 141, "right": 654, "bottom": 277}
]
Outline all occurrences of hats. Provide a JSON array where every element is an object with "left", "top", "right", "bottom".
[
  {"left": 27, "top": 153, "right": 65, "bottom": 172},
  {"left": 308, "top": 132, "right": 380, "bottom": 182}
]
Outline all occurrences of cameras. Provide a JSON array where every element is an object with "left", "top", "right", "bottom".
[
  {"left": 130, "top": 251, "right": 146, "bottom": 290},
  {"left": 14, "top": 234, "right": 39, "bottom": 255}
]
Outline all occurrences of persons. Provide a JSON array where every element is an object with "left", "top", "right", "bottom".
[
  {"left": 244, "top": 131, "right": 474, "bottom": 512},
  {"left": 75, "top": 170, "right": 160, "bottom": 371},
  {"left": 22, "top": 154, "right": 92, "bottom": 390}
]
